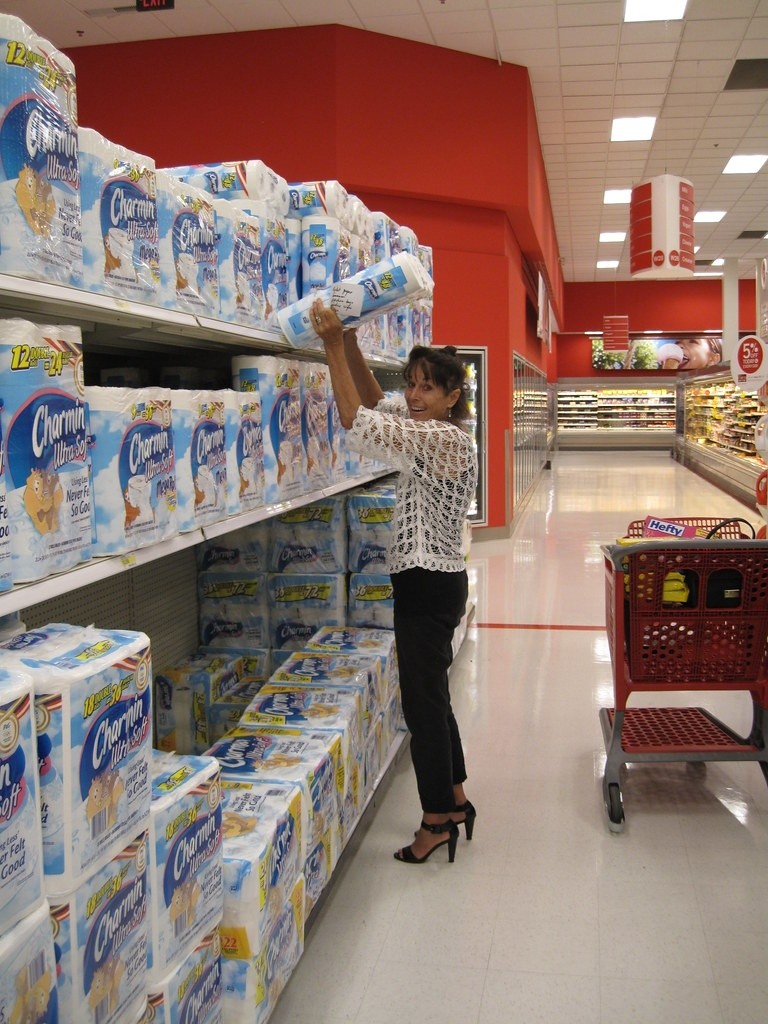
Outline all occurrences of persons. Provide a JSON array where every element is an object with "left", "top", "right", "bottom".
[
  {"left": 308, "top": 296, "right": 479, "bottom": 865},
  {"left": 674, "top": 337, "right": 722, "bottom": 370}
]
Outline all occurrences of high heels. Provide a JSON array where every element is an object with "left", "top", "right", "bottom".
[
  {"left": 413, "top": 799, "right": 476, "bottom": 841},
  {"left": 394, "top": 818, "right": 459, "bottom": 864}
]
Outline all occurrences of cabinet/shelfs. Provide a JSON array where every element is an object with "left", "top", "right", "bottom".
[
  {"left": 0, "top": 267, "right": 474, "bottom": 1024},
  {"left": 557, "top": 390, "right": 675, "bottom": 428},
  {"left": 681, "top": 381, "right": 767, "bottom": 464}
]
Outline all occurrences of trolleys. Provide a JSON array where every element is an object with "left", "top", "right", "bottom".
[{"left": 600, "top": 518, "right": 768, "bottom": 832}]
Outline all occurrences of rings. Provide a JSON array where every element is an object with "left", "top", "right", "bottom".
[{"left": 315, "top": 315, "right": 322, "bottom": 324}]
